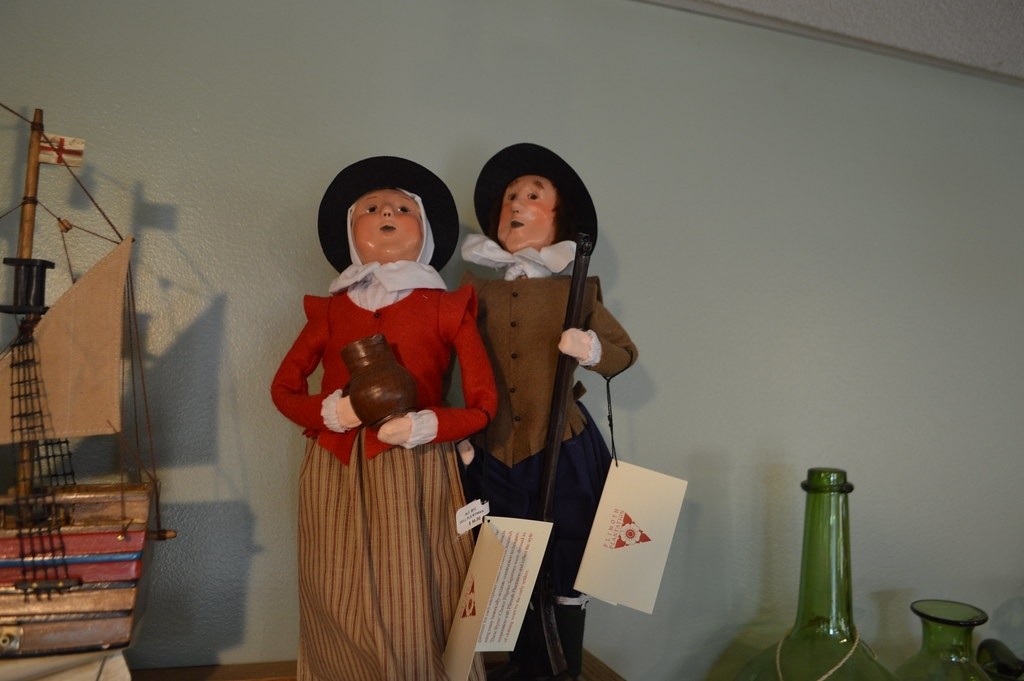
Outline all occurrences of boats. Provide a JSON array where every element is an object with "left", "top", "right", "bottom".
[{"left": 0, "top": 103, "right": 179, "bottom": 658}]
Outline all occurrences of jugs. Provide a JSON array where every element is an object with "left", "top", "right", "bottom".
[{"left": 340, "top": 331, "right": 418, "bottom": 430}]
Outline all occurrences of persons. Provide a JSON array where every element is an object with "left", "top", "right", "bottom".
[{"left": 270, "top": 155, "right": 500, "bottom": 679}]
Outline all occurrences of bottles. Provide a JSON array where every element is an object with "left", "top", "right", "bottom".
[
  {"left": 895, "top": 599, "right": 994, "bottom": 681},
  {"left": 732, "top": 466, "right": 900, "bottom": 680}
]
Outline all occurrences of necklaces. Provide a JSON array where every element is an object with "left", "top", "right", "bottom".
[{"left": 458, "top": 140, "right": 639, "bottom": 681}]
